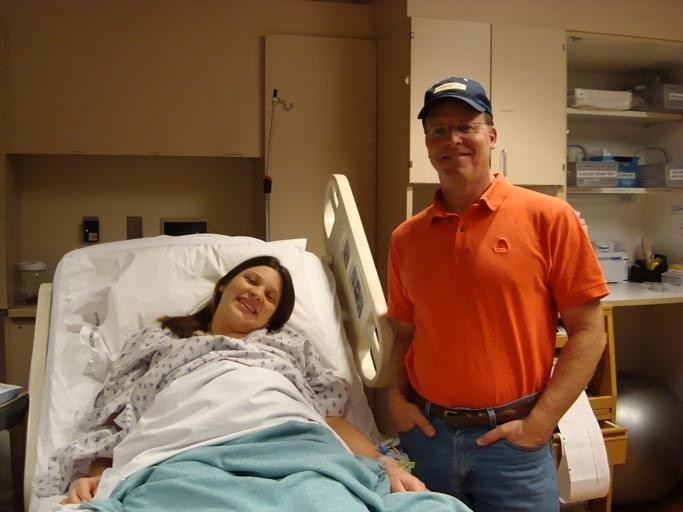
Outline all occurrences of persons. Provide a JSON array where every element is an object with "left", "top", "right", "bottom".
[
  {"left": 37, "top": 255, "right": 474, "bottom": 512},
  {"left": 387, "top": 77, "right": 610, "bottom": 512}
]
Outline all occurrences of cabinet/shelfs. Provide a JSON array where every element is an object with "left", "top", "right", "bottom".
[
  {"left": 551, "top": 304, "right": 628, "bottom": 510},
  {"left": 567, "top": 29, "right": 682, "bottom": 308},
  {"left": 376, "top": 19, "right": 567, "bottom": 304}
]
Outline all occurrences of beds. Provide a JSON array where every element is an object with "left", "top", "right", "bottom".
[{"left": 22, "top": 170, "right": 387, "bottom": 511}]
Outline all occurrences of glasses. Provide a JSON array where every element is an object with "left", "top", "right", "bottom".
[{"left": 424, "top": 122, "right": 493, "bottom": 138}]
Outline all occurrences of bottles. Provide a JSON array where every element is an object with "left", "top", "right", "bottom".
[{"left": 15, "top": 261, "right": 51, "bottom": 310}]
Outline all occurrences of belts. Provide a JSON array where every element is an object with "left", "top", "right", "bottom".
[{"left": 405, "top": 388, "right": 542, "bottom": 429}]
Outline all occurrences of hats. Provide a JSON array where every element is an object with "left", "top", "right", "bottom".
[{"left": 417, "top": 76, "right": 495, "bottom": 121}]
